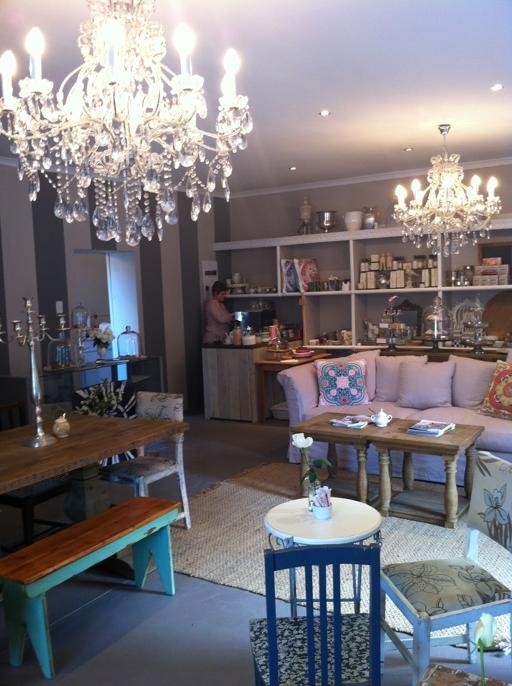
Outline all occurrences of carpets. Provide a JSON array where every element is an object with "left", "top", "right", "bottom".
[{"left": 116, "top": 461, "right": 512, "bottom": 653}]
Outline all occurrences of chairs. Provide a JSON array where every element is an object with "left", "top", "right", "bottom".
[
  {"left": 101, "top": 390, "right": 194, "bottom": 529},
  {"left": 377, "top": 447, "right": 512, "bottom": 685},
  {"left": 250, "top": 540, "right": 380, "bottom": 685}
]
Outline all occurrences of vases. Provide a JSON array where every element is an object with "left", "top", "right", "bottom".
[{"left": 95, "top": 343, "right": 108, "bottom": 364}]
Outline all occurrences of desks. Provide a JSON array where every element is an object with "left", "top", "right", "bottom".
[
  {"left": 264, "top": 495, "right": 383, "bottom": 618},
  {"left": 291, "top": 411, "right": 484, "bottom": 529},
  {"left": 1, "top": 411, "right": 190, "bottom": 556},
  {"left": 44, "top": 354, "right": 166, "bottom": 411}
]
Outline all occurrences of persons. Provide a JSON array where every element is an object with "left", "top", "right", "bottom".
[{"left": 201, "top": 281, "right": 235, "bottom": 344}]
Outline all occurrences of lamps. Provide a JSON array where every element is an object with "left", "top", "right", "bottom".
[
  {"left": 0, "top": 1, "right": 254, "bottom": 248},
  {"left": 393, "top": 125, "right": 502, "bottom": 258}
]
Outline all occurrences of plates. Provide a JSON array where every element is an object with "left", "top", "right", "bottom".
[
  {"left": 292, "top": 350, "right": 314, "bottom": 357},
  {"left": 333, "top": 415, "right": 372, "bottom": 429}
]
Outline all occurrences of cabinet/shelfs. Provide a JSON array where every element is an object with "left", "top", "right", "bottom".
[{"left": 211, "top": 212, "right": 512, "bottom": 351}]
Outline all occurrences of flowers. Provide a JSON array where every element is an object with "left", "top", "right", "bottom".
[{"left": 87, "top": 327, "right": 115, "bottom": 348}]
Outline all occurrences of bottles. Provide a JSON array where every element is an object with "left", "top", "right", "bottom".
[
  {"left": 52, "top": 417, "right": 70, "bottom": 438},
  {"left": 358, "top": 250, "right": 438, "bottom": 288},
  {"left": 233, "top": 327, "right": 241, "bottom": 345}
]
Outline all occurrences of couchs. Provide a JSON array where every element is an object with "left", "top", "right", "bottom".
[{"left": 276, "top": 350, "right": 511, "bottom": 486}]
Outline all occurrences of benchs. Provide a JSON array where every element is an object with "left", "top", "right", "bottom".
[{"left": 0, "top": 493, "right": 184, "bottom": 679}]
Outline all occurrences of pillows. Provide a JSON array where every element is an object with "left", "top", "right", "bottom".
[
  {"left": 395, "top": 359, "right": 455, "bottom": 409},
  {"left": 478, "top": 359, "right": 512, "bottom": 419},
  {"left": 312, "top": 357, "right": 371, "bottom": 406},
  {"left": 448, "top": 353, "right": 497, "bottom": 409},
  {"left": 373, "top": 354, "right": 427, "bottom": 402},
  {"left": 346, "top": 350, "right": 381, "bottom": 401}
]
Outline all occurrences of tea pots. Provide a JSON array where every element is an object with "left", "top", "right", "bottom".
[{"left": 371, "top": 408, "right": 391, "bottom": 428}]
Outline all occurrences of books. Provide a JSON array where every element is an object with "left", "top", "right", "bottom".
[
  {"left": 405, "top": 418, "right": 455, "bottom": 437},
  {"left": 330, "top": 414, "right": 374, "bottom": 430}
]
